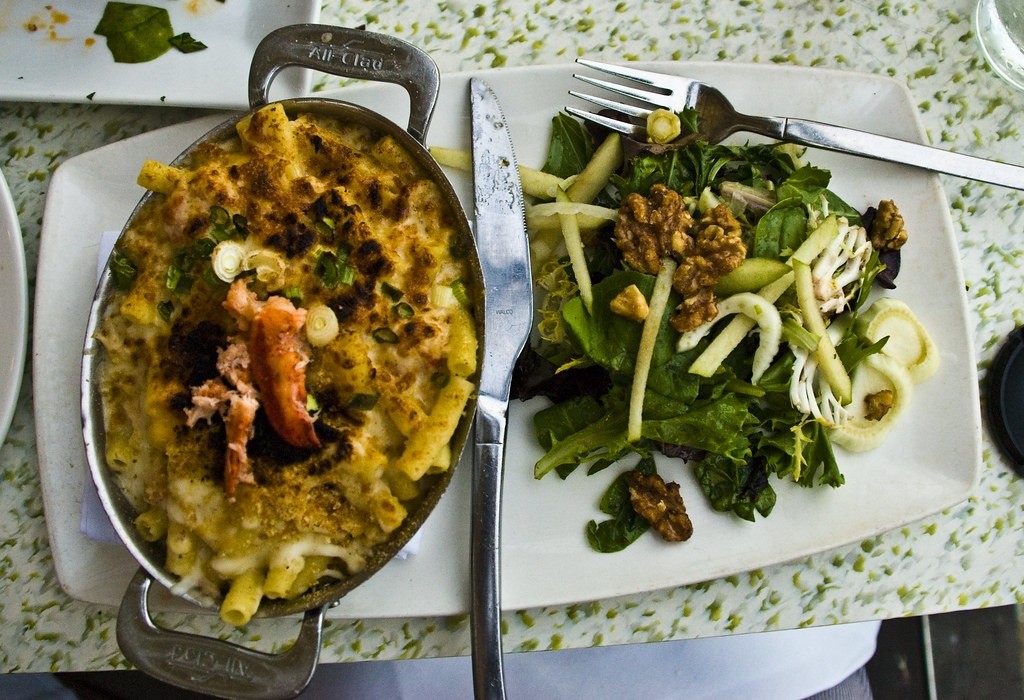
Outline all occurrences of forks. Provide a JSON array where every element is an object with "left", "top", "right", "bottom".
[{"left": 564, "top": 49, "right": 1024, "bottom": 193}]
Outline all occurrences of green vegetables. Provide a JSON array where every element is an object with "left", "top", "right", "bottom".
[{"left": 517, "top": 109, "right": 886, "bottom": 554}]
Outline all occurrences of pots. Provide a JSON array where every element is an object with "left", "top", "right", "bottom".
[{"left": 86, "top": 18, "right": 489, "bottom": 699}]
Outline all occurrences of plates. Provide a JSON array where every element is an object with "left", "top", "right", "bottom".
[
  {"left": 0, "top": 0, "right": 322, "bottom": 116},
  {"left": 35, "top": 38, "right": 980, "bottom": 620},
  {"left": 0, "top": 153, "right": 29, "bottom": 446}
]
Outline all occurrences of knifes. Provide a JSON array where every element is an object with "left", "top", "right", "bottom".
[{"left": 460, "top": 72, "right": 537, "bottom": 696}]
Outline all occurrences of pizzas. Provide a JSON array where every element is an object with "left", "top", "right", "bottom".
[{"left": 91, "top": 101, "right": 480, "bottom": 627}]
